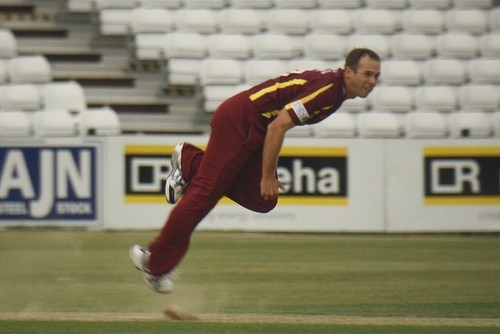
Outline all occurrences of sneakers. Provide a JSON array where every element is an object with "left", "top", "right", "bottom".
[
  {"left": 165, "top": 143, "right": 188, "bottom": 204},
  {"left": 130, "top": 244, "right": 176, "bottom": 293}
]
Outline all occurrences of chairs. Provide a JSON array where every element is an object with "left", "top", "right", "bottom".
[{"left": 0, "top": 0, "right": 500, "bottom": 140}]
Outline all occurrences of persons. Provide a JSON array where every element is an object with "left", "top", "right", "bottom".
[{"left": 128, "top": 48, "right": 381, "bottom": 294}]
